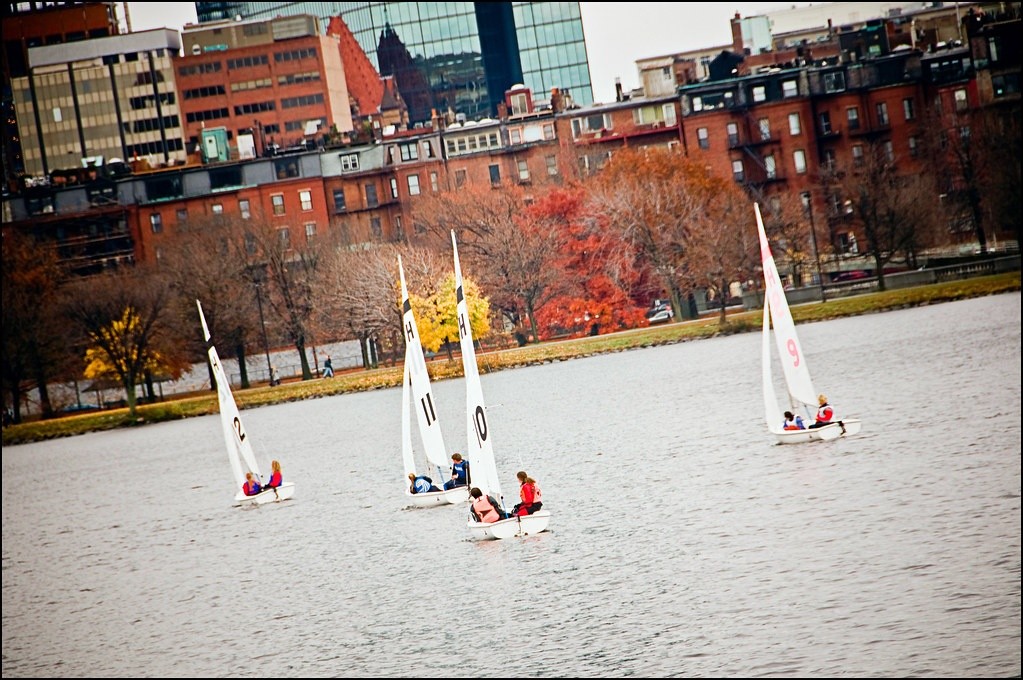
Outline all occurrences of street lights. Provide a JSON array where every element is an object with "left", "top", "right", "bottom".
[
  {"left": 254, "top": 280, "right": 276, "bottom": 387},
  {"left": 798, "top": 190, "right": 826, "bottom": 303}
]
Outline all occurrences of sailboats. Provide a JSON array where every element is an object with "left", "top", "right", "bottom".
[
  {"left": 396, "top": 252, "right": 467, "bottom": 507},
  {"left": 194, "top": 297, "right": 296, "bottom": 505},
  {"left": 753, "top": 200, "right": 863, "bottom": 442},
  {"left": 450, "top": 227, "right": 552, "bottom": 540}
]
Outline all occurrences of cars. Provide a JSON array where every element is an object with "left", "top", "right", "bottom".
[
  {"left": 57, "top": 403, "right": 103, "bottom": 414},
  {"left": 641, "top": 299, "right": 675, "bottom": 324}
]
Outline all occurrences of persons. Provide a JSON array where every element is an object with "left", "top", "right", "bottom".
[
  {"left": 783, "top": 411, "right": 804, "bottom": 429},
  {"left": 263, "top": 461, "right": 282, "bottom": 491},
  {"left": 408, "top": 473, "right": 440, "bottom": 494},
  {"left": 273, "top": 370, "right": 281, "bottom": 385},
  {"left": 323, "top": 356, "right": 334, "bottom": 378},
  {"left": 808, "top": 395, "right": 833, "bottom": 428},
  {"left": 443, "top": 453, "right": 471, "bottom": 491},
  {"left": 470, "top": 487, "right": 514, "bottom": 523},
  {"left": 243, "top": 473, "right": 261, "bottom": 496},
  {"left": 511, "top": 471, "right": 542, "bottom": 514}
]
